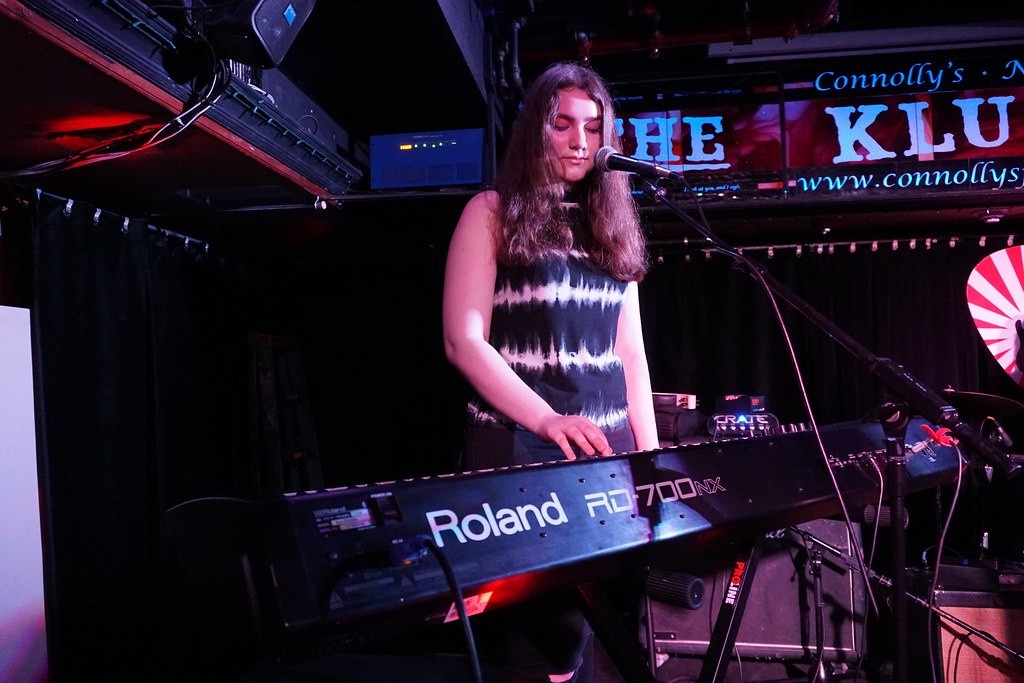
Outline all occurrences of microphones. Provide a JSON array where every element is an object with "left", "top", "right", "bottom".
[
  {"left": 999, "top": 417, "right": 1013, "bottom": 446},
  {"left": 593, "top": 145, "right": 684, "bottom": 183}
]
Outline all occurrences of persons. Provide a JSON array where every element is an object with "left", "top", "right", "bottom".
[{"left": 441, "top": 64, "right": 661, "bottom": 682}]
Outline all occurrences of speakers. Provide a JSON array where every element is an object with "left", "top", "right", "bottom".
[
  {"left": 203, "top": 0, "right": 317, "bottom": 69},
  {"left": 640, "top": 519, "right": 867, "bottom": 662},
  {"left": 870, "top": 590, "right": 1024, "bottom": 683}
]
